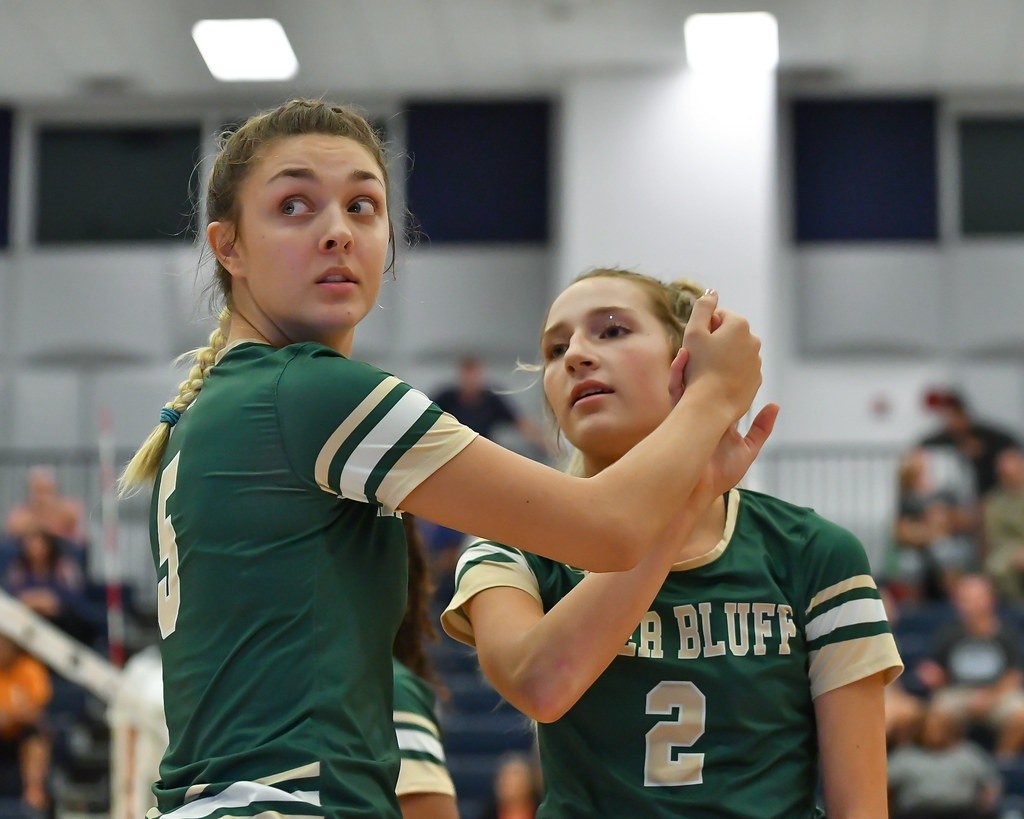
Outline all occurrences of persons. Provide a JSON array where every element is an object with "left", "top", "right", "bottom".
[
  {"left": 0, "top": 358, "right": 1024, "bottom": 819},
  {"left": 115, "top": 100, "right": 765, "bottom": 819},
  {"left": 439, "top": 265, "right": 904, "bottom": 819}
]
